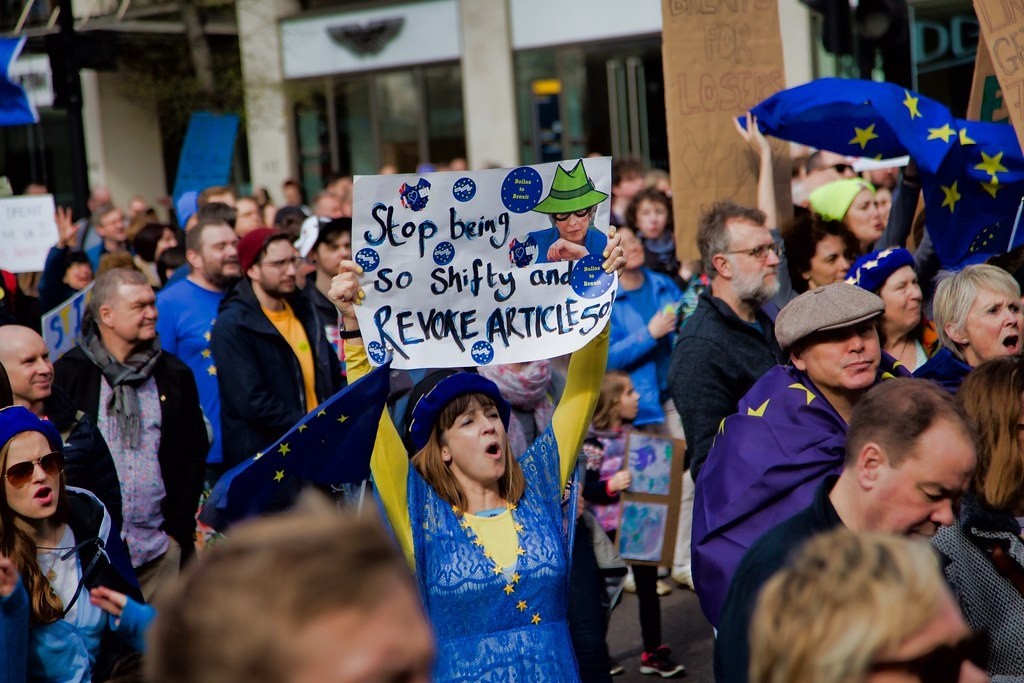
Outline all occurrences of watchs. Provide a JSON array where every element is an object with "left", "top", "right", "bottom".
[{"left": 339, "top": 322, "right": 362, "bottom": 339}]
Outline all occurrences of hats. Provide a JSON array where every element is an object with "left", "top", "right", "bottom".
[
  {"left": 408, "top": 371, "right": 512, "bottom": 450},
  {"left": 235, "top": 226, "right": 291, "bottom": 274},
  {"left": 844, "top": 244, "right": 915, "bottom": 297},
  {"left": 0, "top": 405, "right": 64, "bottom": 453},
  {"left": 774, "top": 280, "right": 886, "bottom": 350},
  {"left": 807, "top": 178, "right": 878, "bottom": 222}
]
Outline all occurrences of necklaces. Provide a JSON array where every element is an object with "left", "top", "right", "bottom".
[
  {"left": 36, "top": 529, "right": 66, "bottom": 581},
  {"left": 898, "top": 336, "right": 911, "bottom": 362}
]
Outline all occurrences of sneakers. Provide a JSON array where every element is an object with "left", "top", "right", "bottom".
[
  {"left": 608, "top": 655, "right": 624, "bottom": 676},
  {"left": 640, "top": 645, "right": 685, "bottom": 678}
]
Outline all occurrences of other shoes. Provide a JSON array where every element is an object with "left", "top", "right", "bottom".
[
  {"left": 655, "top": 580, "right": 674, "bottom": 594},
  {"left": 672, "top": 565, "right": 695, "bottom": 589},
  {"left": 622, "top": 575, "right": 637, "bottom": 592}
]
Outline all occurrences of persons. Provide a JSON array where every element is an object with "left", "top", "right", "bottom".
[{"left": 0, "top": 111, "right": 1024, "bottom": 683}]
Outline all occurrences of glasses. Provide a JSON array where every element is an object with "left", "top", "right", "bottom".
[
  {"left": 807, "top": 163, "right": 856, "bottom": 173},
  {"left": 718, "top": 241, "right": 782, "bottom": 261},
  {"left": 3, "top": 451, "right": 67, "bottom": 489},
  {"left": 257, "top": 256, "right": 305, "bottom": 273},
  {"left": 867, "top": 627, "right": 995, "bottom": 683}
]
